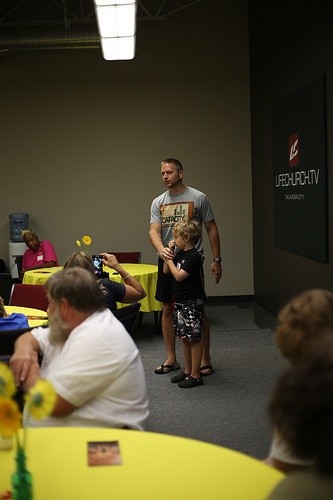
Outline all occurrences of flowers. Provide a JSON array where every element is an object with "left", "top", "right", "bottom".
[
  {"left": 76, "top": 235, "right": 91, "bottom": 254},
  {"left": 0, "top": 363, "right": 58, "bottom": 449}
]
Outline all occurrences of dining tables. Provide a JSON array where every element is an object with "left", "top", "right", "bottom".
[
  {"left": 0, "top": 305, "right": 48, "bottom": 335},
  {"left": 0, "top": 425, "right": 288, "bottom": 500},
  {"left": 21, "top": 262, "right": 162, "bottom": 328}
]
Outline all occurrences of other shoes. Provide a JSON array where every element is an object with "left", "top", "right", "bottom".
[
  {"left": 172, "top": 369, "right": 187, "bottom": 383},
  {"left": 201, "top": 360, "right": 212, "bottom": 375},
  {"left": 155, "top": 359, "right": 179, "bottom": 373},
  {"left": 178, "top": 375, "right": 203, "bottom": 389}
]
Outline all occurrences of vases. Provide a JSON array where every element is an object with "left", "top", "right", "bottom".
[{"left": 11, "top": 446, "right": 34, "bottom": 500}]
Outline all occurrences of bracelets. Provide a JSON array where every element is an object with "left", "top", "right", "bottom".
[
  {"left": 122, "top": 273, "right": 130, "bottom": 280},
  {"left": 212, "top": 257, "right": 222, "bottom": 262}
]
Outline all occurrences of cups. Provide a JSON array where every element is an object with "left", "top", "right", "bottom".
[{"left": 0, "top": 423, "right": 14, "bottom": 450}]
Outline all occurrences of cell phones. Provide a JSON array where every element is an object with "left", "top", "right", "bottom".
[{"left": 92, "top": 255, "right": 103, "bottom": 279}]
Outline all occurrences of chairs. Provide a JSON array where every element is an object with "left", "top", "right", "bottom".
[
  {"left": 8, "top": 283, "right": 52, "bottom": 313},
  {"left": 15, "top": 256, "right": 24, "bottom": 279},
  {"left": 104, "top": 251, "right": 141, "bottom": 264}
]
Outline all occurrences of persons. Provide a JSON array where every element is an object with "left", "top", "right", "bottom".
[
  {"left": 0, "top": 297, "right": 29, "bottom": 330},
  {"left": 162, "top": 219, "right": 204, "bottom": 389},
  {"left": 64, "top": 252, "right": 147, "bottom": 311},
  {"left": 148, "top": 159, "right": 223, "bottom": 378},
  {"left": 21, "top": 229, "right": 59, "bottom": 282},
  {"left": 263, "top": 288, "right": 333, "bottom": 477},
  {"left": 261, "top": 354, "right": 333, "bottom": 500},
  {"left": 10, "top": 266, "right": 149, "bottom": 434}
]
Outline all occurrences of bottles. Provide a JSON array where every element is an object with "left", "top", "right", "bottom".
[{"left": 8, "top": 213, "right": 29, "bottom": 242}]
